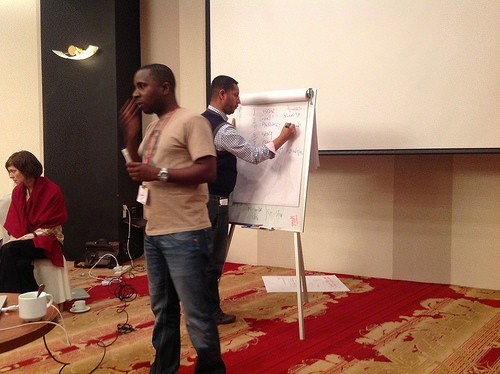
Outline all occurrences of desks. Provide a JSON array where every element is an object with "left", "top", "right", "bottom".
[{"left": 0, "top": 293, "right": 59, "bottom": 354}]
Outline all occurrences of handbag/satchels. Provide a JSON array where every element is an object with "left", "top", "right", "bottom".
[{"left": 74, "top": 238, "right": 121, "bottom": 268}]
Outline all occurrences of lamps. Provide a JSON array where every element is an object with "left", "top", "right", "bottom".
[{"left": 52, "top": 45, "right": 98, "bottom": 60}]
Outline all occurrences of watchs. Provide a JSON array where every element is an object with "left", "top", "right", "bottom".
[{"left": 157, "top": 168, "right": 169, "bottom": 183}]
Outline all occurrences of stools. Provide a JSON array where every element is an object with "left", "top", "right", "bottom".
[{"left": 32, "top": 255, "right": 71, "bottom": 313}]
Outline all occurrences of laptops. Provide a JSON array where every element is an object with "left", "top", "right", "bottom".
[{"left": 70, "top": 287, "right": 90, "bottom": 299}]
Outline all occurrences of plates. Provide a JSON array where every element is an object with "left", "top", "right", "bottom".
[{"left": 70, "top": 306, "right": 91, "bottom": 313}]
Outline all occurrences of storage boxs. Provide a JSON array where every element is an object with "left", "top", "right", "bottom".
[{"left": 85, "top": 241, "right": 118, "bottom": 266}]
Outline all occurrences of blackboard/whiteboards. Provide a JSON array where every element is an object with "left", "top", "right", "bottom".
[{"left": 228, "top": 89, "right": 318, "bottom": 233}]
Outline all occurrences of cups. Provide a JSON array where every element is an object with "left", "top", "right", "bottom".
[
  {"left": 18, "top": 291, "right": 54, "bottom": 322},
  {"left": 72, "top": 300, "right": 85, "bottom": 310}
]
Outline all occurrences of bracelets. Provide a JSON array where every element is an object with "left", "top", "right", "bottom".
[{"left": 32, "top": 231, "right": 38, "bottom": 238}]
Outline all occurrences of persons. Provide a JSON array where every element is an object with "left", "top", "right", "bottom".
[
  {"left": 200, "top": 75, "right": 297, "bottom": 326},
  {"left": 120, "top": 62, "right": 227, "bottom": 374},
  {"left": 0, "top": 150, "right": 69, "bottom": 294}
]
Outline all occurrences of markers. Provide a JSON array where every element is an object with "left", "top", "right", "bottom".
[
  {"left": 284, "top": 125, "right": 290, "bottom": 128},
  {"left": 259, "top": 226, "right": 273, "bottom": 230},
  {"left": 246, "top": 223, "right": 262, "bottom": 227}
]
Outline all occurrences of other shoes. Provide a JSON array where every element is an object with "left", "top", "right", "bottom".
[{"left": 218, "top": 312, "right": 236, "bottom": 324}]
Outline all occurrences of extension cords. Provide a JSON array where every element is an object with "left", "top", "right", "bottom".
[{"left": 114, "top": 265, "right": 132, "bottom": 276}]
